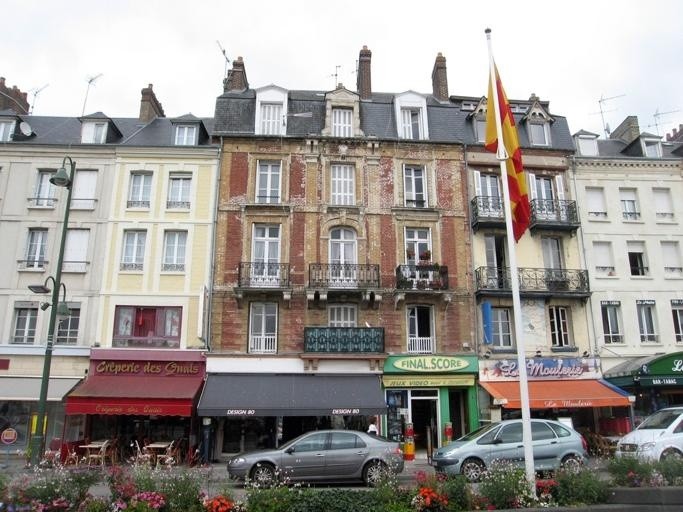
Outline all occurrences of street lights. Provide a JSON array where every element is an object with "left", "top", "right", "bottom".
[{"left": 29, "top": 157, "right": 75, "bottom": 469}]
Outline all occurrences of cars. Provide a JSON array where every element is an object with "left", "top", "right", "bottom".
[
  {"left": 227, "top": 430, "right": 403, "bottom": 487},
  {"left": 615, "top": 407, "right": 683, "bottom": 466},
  {"left": 433, "top": 418, "right": 588, "bottom": 481}
]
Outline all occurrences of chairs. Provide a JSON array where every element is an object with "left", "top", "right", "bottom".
[
  {"left": 591, "top": 434, "right": 618, "bottom": 458},
  {"left": 64, "top": 438, "right": 182, "bottom": 471}
]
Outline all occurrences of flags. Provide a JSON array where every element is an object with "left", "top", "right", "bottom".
[{"left": 483, "top": 54, "right": 531, "bottom": 244}]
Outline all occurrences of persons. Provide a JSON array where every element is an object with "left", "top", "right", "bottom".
[{"left": 366, "top": 416, "right": 379, "bottom": 436}]
